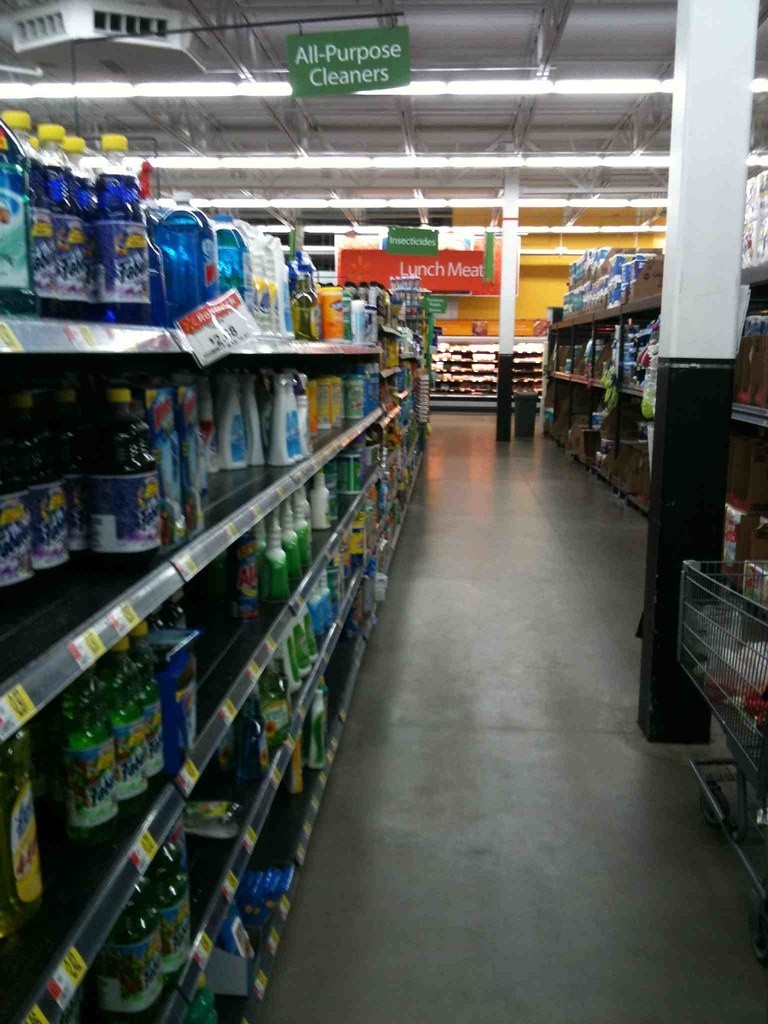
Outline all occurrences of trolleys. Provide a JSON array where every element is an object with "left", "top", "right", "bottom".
[{"left": 674, "top": 561, "right": 768, "bottom": 966}]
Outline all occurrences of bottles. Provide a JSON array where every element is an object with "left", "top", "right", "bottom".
[
  {"left": 291, "top": 273, "right": 320, "bottom": 341},
  {"left": 307, "top": 689, "right": 326, "bottom": 769},
  {"left": 0, "top": 109, "right": 95, "bottom": 322},
  {"left": 0, "top": 388, "right": 91, "bottom": 603},
  {"left": 144, "top": 191, "right": 218, "bottom": 330},
  {"left": 316, "top": 677, "right": 327, "bottom": 737},
  {"left": 257, "top": 659, "right": 290, "bottom": 749},
  {"left": 95, "top": 133, "right": 150, "bottom": 325},
  {"left": 234, "top": 689, "right": 271, "bottom": 785},
  {"left": 215, "top": 213, "right": 254, "bottom": 316},
  {"left": 308, "top": 374, "right": 365, "bottom": 434},
  {"left": 85, "top": 388, "right": 161, "bottom": 568},
  {"left": 48, "top": 621, "right": 166, "bottom": 848},
  {"left": 89, "top": 841, "right": 192, "bottom": 1019}
]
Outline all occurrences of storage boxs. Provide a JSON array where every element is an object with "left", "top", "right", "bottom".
[{"left": 547, "top": 169, "right": 768, "bottom": 605}]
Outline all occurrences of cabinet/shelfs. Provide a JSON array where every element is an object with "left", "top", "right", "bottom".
[
  {"left": 1, "top": 314, "right": 432, "bottom": 1024},
  {"left": 545, "top": 263, "right": 767, "bottom": 583},
  {"left": 429, "top": 344, "right": 544, "bottom": 409}
]
